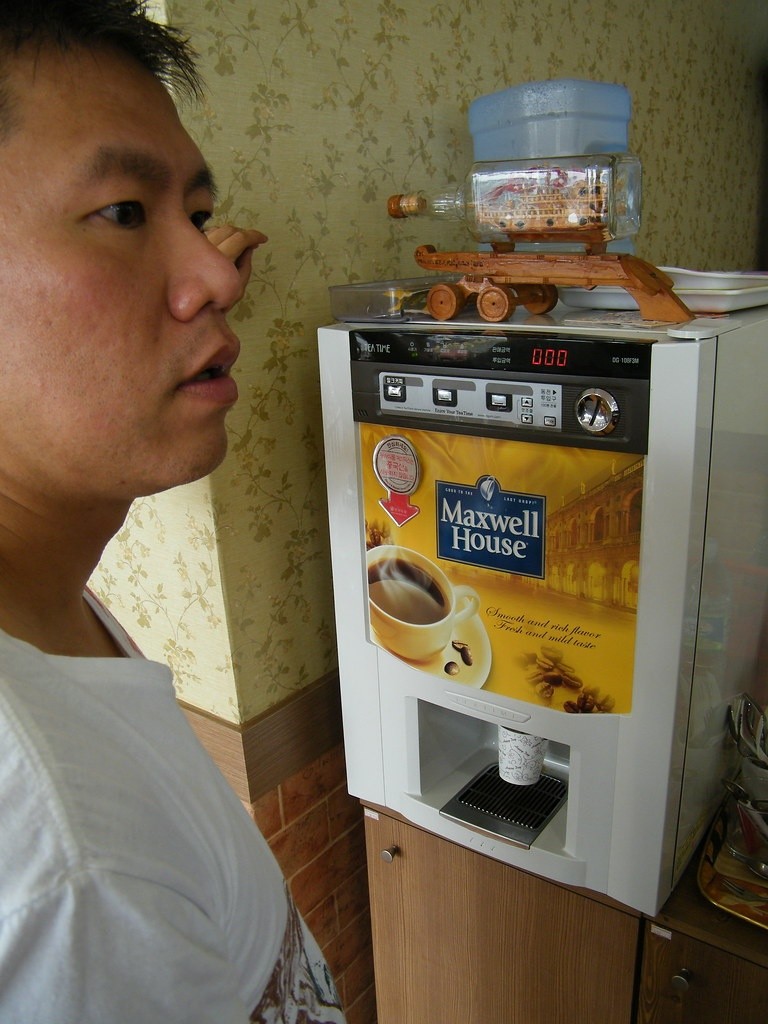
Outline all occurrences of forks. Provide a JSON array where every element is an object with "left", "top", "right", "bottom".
[{"left": 719, "top": 876, "right": 768, "bottom": 902}]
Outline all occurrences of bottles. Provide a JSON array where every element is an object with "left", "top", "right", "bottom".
[{"left": 384, "top": 155, "right": 650, "bottom": 249}]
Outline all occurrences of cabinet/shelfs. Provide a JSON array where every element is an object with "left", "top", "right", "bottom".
[{"left": 357, "top": 798, "right": 768, "bottom": 1024}]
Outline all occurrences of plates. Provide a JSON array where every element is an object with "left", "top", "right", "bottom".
[{"left": 367, "top": 583, "right": 492, "bottom": 690}]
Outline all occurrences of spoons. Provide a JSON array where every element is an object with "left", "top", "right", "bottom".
[{"left": 747, "top": 862, "right": 768, "bottom": 880}]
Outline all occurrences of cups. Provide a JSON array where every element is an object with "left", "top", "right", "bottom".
[
  {"left": 726, "top": 776, "right": 768, "bottom": 865},
  {"left": 365, "top": 544, "right": 481, "bottom": 661},
  {"left": 496, "top": 724, "right": 549, "bottom": 787}
]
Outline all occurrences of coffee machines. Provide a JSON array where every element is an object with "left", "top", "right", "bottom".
[{"left": 315, "top": 269, "right": 768, "bottom": 919}]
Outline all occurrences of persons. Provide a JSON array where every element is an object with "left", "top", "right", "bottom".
[{"left": 1, "top": 0, "right": 356, "bottom": 1023}]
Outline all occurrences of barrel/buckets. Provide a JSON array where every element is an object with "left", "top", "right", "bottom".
[{"left": 467, "top": 80, "right": 635, "bottom": 257}]
